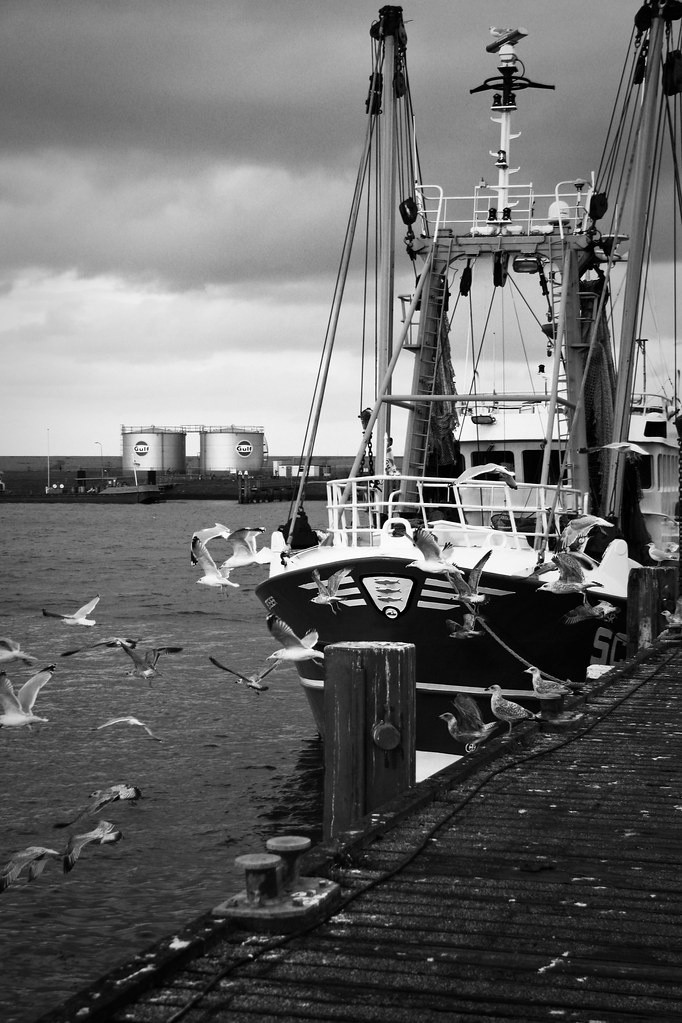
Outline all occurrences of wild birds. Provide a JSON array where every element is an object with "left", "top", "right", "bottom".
[
  {"left": 60, "top": 820, "right": 124, "bottom": 874},
  {"left": 449, "top": 442, "right": 682, "bottom": 624},
  {"left": 42, "top": 593, "right": 100, "bottom": 628},
  {"left": 439, "top": 549, "right": 621, "bottom": 748},
  {"left": 190, "top": 523, "right": 232, "bottom": 566},
  {"left": 266, "top": 612, "right": 324, "bottom": 661},
  {"left": 90, "top": 716, "right": 161, "bottom": 741},
  {"left": 405, "top": 525, "right": 465, "bottom": 575},
  {"left": 0, "top": 846, "right": 62, "bottom": 892},
  {"left": 53, "top": 784, "right": 142, "bottom": 829},
  {"left": 60, "top": 636, "right": 142, "bottom": 656},
  {"left": 0, "top": 662, "right": 57, "bottom": 730},
  {"left": 191, "top": 536, "right": 239, "bottom": 598},
  {"left": 219, "top": 527, "right": 271, "bottom": 568},
  {"left": 119, "top": 641, "right": 183, "bottom": 688},
  {"left": 0, "top": 636, "right": 38, "bottom": 666},
  {"left": 310, "top": 566, "right": 353, "bottom": 615},
  {"left": 209, "top": 657, "right": 283, "bottom": 695}
]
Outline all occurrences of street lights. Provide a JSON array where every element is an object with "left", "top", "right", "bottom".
[{"left": 95, "top": 442, "right": 103, "bottom": 477}]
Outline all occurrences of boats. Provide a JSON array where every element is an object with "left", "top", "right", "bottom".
[{"left": 255, "top": 1, "right": 682, "bottom": 786}]
